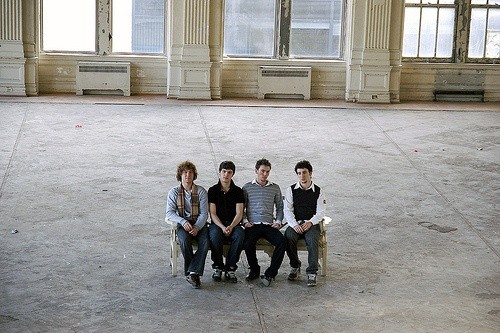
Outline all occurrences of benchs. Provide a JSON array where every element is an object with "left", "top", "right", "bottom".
[
  {"left": 432, "top": 69, "right": 487, "bottom": 102},
  {"left": 169, "top": 217, "right": 328, "bottom": 277}
]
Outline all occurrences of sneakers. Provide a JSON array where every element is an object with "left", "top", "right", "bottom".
[
  {"left": 288, "top": 267, "right": 300, "bottom": 280},
  {"left": 307, "top": 274, "right": 317, "bottom": 287}
]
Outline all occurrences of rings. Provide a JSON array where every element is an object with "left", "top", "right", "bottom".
[{"left": 304, "top": 229, "right": 307, "bottom": 231}]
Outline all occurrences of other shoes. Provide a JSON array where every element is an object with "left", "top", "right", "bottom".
[
  {"left": 259, "top": 274, "right": 272, "bottom": 287},
  {"left": 224, "top": 270, "right": 237, "bottom": 283},
  {"left": 212, "top": 269, "right": 222, "bottom": 282},
  {"left": 245, "top": 269, "right": 260, "bottom": 281},
  {"left": 186, "top": 274, "right": 201, "bottom": 288}
]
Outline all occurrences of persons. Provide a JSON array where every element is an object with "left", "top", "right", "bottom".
[
  {"left": 242, "top": 158, "right": 287, "bottom": 287},
  {"left": 284, "top": 159, "right": 327, "bottom": 287},
  {"left": 165, "top": 160, "right": 210, "bottom": 289},
  {"left": 208, "top": 161, "right": 245, "bottom": 282}
]
